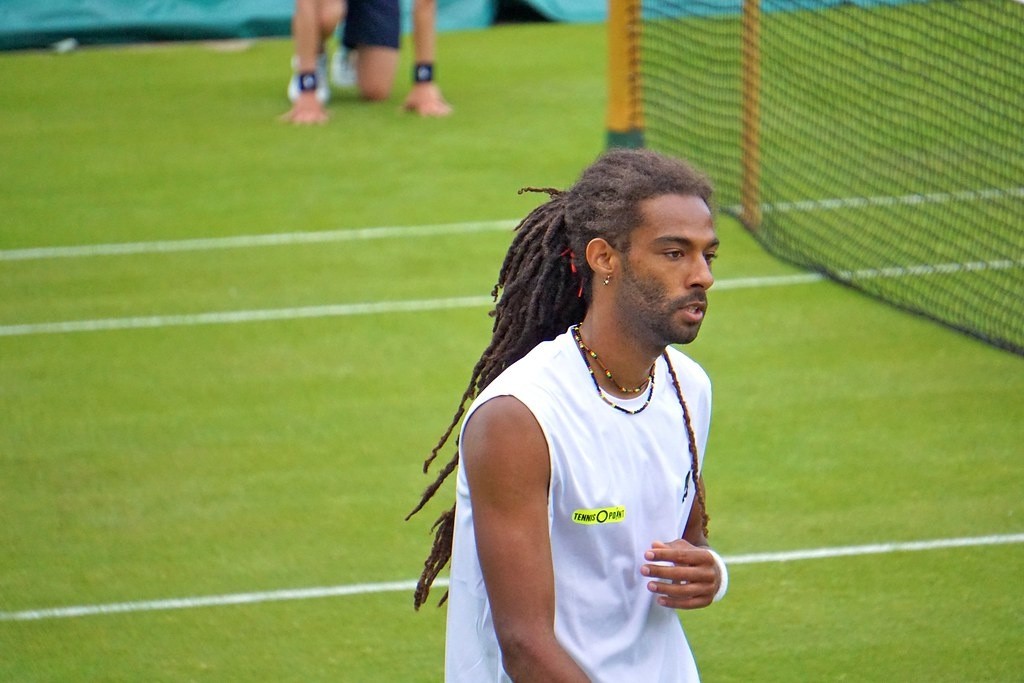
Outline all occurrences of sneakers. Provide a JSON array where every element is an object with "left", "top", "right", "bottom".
[
  {"left": 289, "top": 53, "right": 331, "bottom": 105},
  {"left": 332, "top": 41, "right": 360, "bottom": 86}
]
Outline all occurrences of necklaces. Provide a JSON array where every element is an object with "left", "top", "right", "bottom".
[{"left": 572, "top": 322, "right": 656, "bottom": 415}]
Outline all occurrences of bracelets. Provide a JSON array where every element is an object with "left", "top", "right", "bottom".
[
  {"left": 679, "top": 549, "right": 729, "bottom": 602},
  {"left": 414, "top": 62, "right": 434, "bottom": 83},
  {"left": 299, "top": 70, "right": 317, "bottom": 92}
]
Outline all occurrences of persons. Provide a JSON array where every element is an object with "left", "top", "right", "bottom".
[
  {"left": 280, "top": 0, "right": 452, "bottom": 124},
  {"left": 405, "top": 147, "right": 729, "bottom": 683}
]
